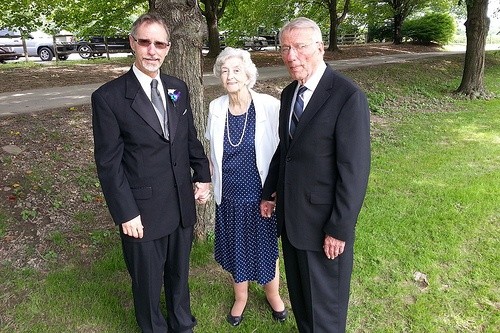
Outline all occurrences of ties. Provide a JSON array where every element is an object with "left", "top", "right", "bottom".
[
  {"left": 150, "top": 79, "right": 165, "bottom": 121},
  {"left": 289, "top": 85, "right": 308, "bottom": 146}
]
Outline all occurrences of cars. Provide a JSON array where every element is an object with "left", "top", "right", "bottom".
[
  {"left": 74, "top": 27, "right": 135, "bottom": 59},
  {"left": 218, "top": 29, "right": 270, "bottom": 51},
  {"left": 0, "top": 21, "right": 75, "bottom": 61}
]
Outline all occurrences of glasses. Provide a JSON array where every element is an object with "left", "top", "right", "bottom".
[
  {"left": 276, "top": 41, "right": 324, "bottom": 54},
  {"left": 131, "top": 35, "right": 169, "bottom": 50}
]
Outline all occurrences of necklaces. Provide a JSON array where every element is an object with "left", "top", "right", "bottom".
[{"left": 226, "top": 106, "right": 248, "bottom": 147}]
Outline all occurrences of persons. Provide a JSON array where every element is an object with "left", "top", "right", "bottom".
[
  {"left": 91, "top": 10, "right": 211, "bottom": 333},
  {"left": 260, "top": 17, "right": 371, "bottom": 333},
  {"left": 193, "top": 46, "right": 287, "bottom": 325}
]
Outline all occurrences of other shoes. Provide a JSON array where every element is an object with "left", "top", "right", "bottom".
[
  {"left": 265, "top": 298, "right": 288, "bottom": 322},
  {"left": 227, "top": 308, "right": 243, "bottom": 326}
]
